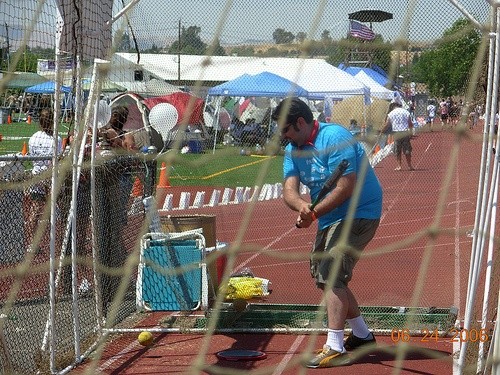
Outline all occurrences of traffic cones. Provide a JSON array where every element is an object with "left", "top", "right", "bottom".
[
  {"left": 6, "top": 115, "right": 11, "bottom": 124},
  {"left": 156, "top": 162, "right": 172, "bottom": 190},
  {"left": 129, "top": 177, "right": 143, "bottom": 199},
  {"left": 61, "top": 138, "right": 67, "bottom": 150},
  {"left": 372, "top": 145, "right": 380, "bottom": 156},
  {"left": 386, "top": 134, "right": 392, "bottom": 145},
  {"left": 26, "top": 116, "right": 31, "bottom": 125},
  {"left": 21, "top": 142, "right": 28, "bottom": 156}
]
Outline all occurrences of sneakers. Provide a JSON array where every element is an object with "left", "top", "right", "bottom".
[
  {"left": 304, "top": 344, "right": 349, "bottom": 367},
  {"left": 343, "top": 330, "right": 377, "bottom": 352}
]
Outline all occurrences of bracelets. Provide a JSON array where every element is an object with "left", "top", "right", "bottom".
[{"left": 312, "top": 211, "right": 316, "bottom": 221}]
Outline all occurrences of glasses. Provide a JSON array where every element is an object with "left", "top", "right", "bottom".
[{"left": 281, "top": 117, "right": 297, "bottom": 134}]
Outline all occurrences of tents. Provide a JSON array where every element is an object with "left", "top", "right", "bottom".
[
  {"left": 206, "top": 72, "right": 298, "bottom": 155},
  {"left": 19, "top": 80, "right": 203, "bottom": 139},
  {"left": 0, "top": 74, "right": 51, "bottom": 94},
  {"left": 253, "top": 62, "right": 409, "bottom": 138}
]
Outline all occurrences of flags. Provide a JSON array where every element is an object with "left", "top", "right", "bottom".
[{"left": 349, "top": 21, "right": 377, "bottom": 42}]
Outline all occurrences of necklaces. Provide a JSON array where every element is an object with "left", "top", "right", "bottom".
[{"left": 27, "top": 107, "right": 136, "bottom": 302}]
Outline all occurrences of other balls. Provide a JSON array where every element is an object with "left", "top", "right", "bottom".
[{"left": 240, "top": 149, "right": 246, "bottom": 156}]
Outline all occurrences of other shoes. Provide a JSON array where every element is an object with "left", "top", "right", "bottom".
[
  {"left": 76, "top": 279, "right": 91, "bottom": 293},
  {"left": 394, "top": 166, "right": 402, "bottom": 170}
]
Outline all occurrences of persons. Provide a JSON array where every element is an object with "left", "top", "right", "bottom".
[
  {"left": 229, "top": 117, "right": 276, "bottom": 146},
  {"left": 425, "top": 98, "right": 500, "bottom": 134},
  {"left": 408, "top": 105, "right": 418, "bottom": 137},
  {"left": 382, "top": 103, "right": 417, "bottom": 146},
  {"left": 493, "top": 110, "right": 498, "bottom": 154},
  {"left": 382, "top": 102, "right": 414, "bottom": 171},
  {"left": 273, "top": 98, "right": 382, "bottom": 368},
  {"left": 5, "top": 92, "right": 52, "bottom": 116}
]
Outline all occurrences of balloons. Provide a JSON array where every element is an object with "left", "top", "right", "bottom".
[
  {"left": 83, "top": 101, "right": 112, "bottom": 133},
  {"left": 148, "top": 103, "right": 179, "bottom": 141}
]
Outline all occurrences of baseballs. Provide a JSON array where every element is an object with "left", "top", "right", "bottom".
[
  {"left": 294, "top": 158, "right": 350, "bottom": 230},
  {"left": 138, "top": 332, "right": 153, "bottom": 345}
]
